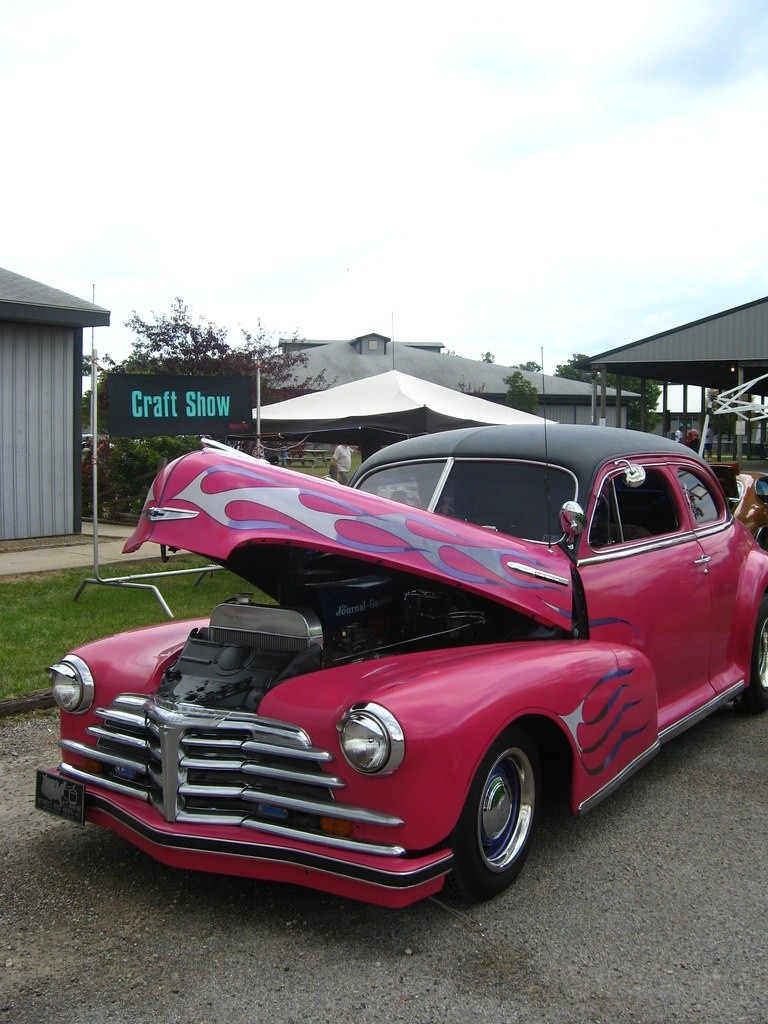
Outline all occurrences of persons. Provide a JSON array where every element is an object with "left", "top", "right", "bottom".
[
  {"left": 675, "top": 422, "right": 714, "bottom": 462},
  {"left": 331, "top": 443, "right": 360, "bottom": 487}
]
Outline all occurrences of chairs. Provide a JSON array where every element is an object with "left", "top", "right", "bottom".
[{"left": 590, "top": 522, "right": 652, "bottom": 548}]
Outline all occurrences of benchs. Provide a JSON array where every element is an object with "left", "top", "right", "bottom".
[
  {"left": 282, "top": 457, "right": 319, "bottom": 462},
  {"left": 467, "top": 483, "right": 673, "bottom": 535},
  {"left": 307, "top": 457, "right": 332, "bottom": 460}
]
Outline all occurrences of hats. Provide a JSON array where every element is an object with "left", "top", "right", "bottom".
[{"left": 687, "top": 429, "right": 699, "bottom": 437}]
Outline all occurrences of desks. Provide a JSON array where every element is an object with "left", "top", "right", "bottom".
[{"left": 287, "top": 450, "right": 328, "bottom": 469}]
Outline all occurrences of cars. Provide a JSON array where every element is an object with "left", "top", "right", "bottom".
[{"left": 33, "top": 424, "right": 767, "bottom": 909}]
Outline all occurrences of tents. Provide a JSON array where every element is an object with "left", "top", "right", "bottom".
[{"left": 252, "top": 368, "right": 561, "bottom": 494}]
